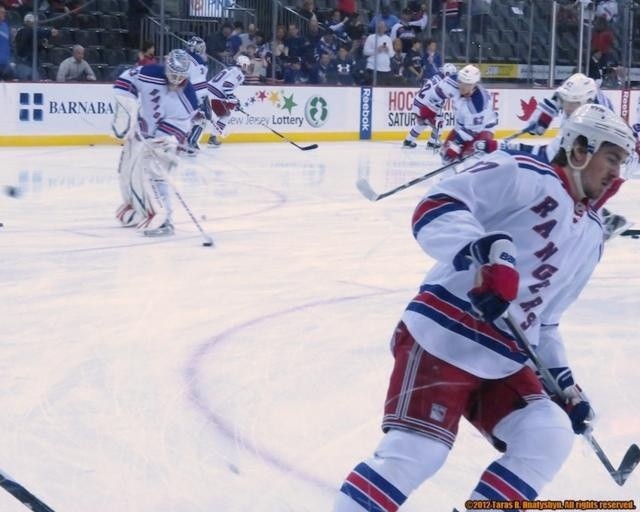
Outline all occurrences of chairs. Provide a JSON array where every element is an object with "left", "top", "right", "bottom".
[
  {"left": 299, "top": 0, "right": 640, "bottom": 74},
  {"left": 1, "top": 0, "right": 159, "bottom": 88}
]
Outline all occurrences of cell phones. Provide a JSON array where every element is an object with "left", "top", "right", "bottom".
[{"left": 383, "top": 42, "right": 386, "bottom": 47}]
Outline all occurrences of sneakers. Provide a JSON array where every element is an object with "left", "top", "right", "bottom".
[
  {"left": 403, "top": 140, "right": 416, "bottom": 147},
  {"left": 428, "top": 141, "right": 441, "bottom": 148},
  {"left": 208, "top": 136, "right": 221, "bottom": 145}
]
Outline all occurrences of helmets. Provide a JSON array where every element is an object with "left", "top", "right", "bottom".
[
  {"left": 187, "top": 36, "right": 206, "bottom": 54},
  {"left": 236, "top": 55, "right": 251, "bottom": 73},
  {"left": 165, "top": 48, "right": 191, "bottom": 85},
  {"left": 457, "top": 64, "right": 480, "bottom": 83},
  {"left": 560, "top": 104, "right": 635, "bottom": 170},
  {"left": 560, "top": 72, "right": 596, "bottom": 102},
  {"left": 443, "top": 63, "right": 456, "bottom": 76}
]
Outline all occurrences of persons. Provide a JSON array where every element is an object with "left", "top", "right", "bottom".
[
  {"left": 113, "top": 50, "right": 201, "bottom": 233},
  {"left": 0, "top": 4, "right": 15, "bottom": 79},
  {"left": 504, "top": 65, "right": 639, "bottom": 238},
  {"left": 139, "top": 40, "right": 157, "bottom": 66},
  {"left": 422, "top": 67, "right": 495, "bottom": 169},
  {"left": 56, "top": 43, "right": 97, "bottom": 83},
  {"left": 206, "top": 0, "right": 491, "bottom": 89},
  {"left": 407, "top": 64, "right": 456, "bottom": 146},
  {"left": 182, "top": 36, "right": 210, "bottom": 150},
  {"left": 15, "top": 12, "right": 57, "bottom": 80},
  {"left": 590, "top": 13, "right": 615, "bottom": 79},
  {"left": 555, "top": 0, "right": 618, "bottom": 27},
  {"left": 204, "top": 53, "right": 252, "bottom": 145},
  {"left": 0, "top": 0, "right": 82, "bottom": 24},
  {"left": 331, "top": 103, "right": 637, "bottom": 508}
]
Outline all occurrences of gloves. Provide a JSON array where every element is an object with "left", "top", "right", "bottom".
[
  {"left": 467, "top": 231, "right": 520, "bottom": 321},
  {"left": 538, "top": 368, "right": 595, "bottom": 434}
]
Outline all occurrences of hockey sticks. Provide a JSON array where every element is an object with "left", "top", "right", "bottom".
[
  {"left": 433, "top": 123, "right": 440, "bottom": 155},
  {"left": 235, "top": 107, "right": 317, "bottom": 150},
  {"left": 135, "top": 130, "right": 212, "bottom": 246},
  {"left": 355, "top": 124, "right": 536, "bottom": 202},
  {"left": 501, "top": 311, "right": 639, "bottom": 485}
]
